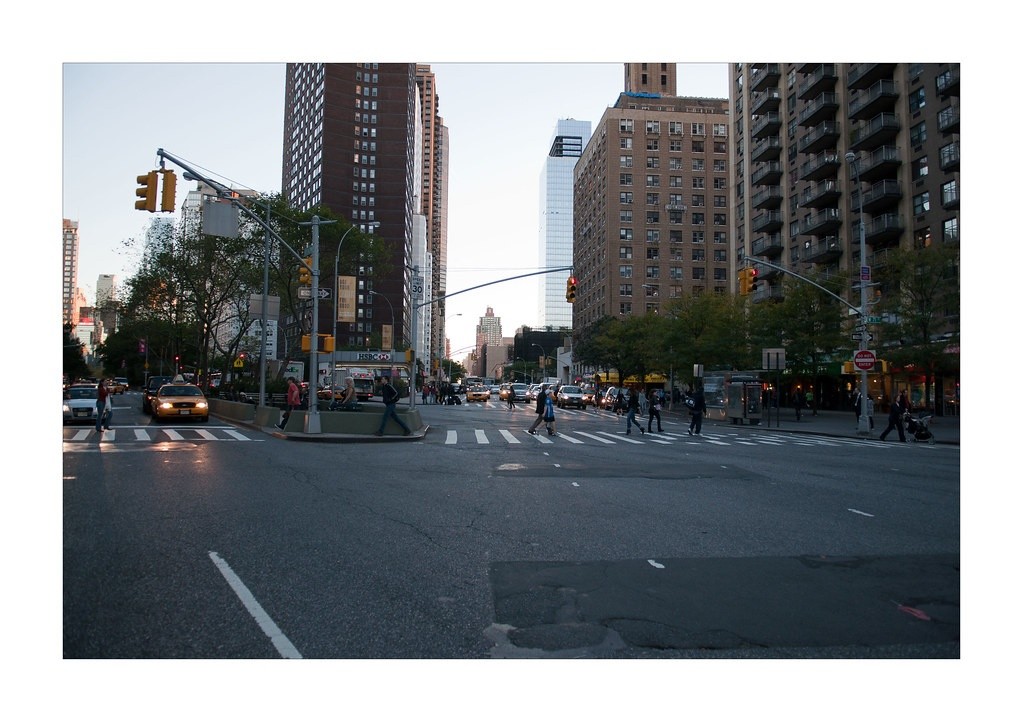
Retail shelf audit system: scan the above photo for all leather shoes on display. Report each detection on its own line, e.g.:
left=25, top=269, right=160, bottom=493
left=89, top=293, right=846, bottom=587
left=96, top=428, right=104, bottom=433
left=104, top=426, right=112, bottom=430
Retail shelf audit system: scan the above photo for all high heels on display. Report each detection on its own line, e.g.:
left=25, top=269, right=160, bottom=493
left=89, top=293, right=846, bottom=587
left=640, top=427, right=646, bottom=436
left=624, top=429, right=632, bottom=437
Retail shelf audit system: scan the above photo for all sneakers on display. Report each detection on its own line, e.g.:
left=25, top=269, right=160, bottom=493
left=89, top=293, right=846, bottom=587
left=274, top=423, right=284, bottom=431
left=372, top=429, right=383, bottom=436
left=403, top=430, right=413, bottom=436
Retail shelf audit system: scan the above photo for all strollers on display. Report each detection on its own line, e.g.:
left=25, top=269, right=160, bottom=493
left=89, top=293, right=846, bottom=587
left=905, top=410, right=936, bottom=446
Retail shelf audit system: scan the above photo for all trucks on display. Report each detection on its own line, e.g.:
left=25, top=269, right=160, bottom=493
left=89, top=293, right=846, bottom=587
left=326, top=367, right=376, bottom=401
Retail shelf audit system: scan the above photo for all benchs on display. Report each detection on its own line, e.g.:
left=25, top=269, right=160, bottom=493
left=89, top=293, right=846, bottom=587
left=244, top=392, right=271, bottom=404
left=272, top=393, right=287, bottom=403
left=218, top=391, right=239, bottom=401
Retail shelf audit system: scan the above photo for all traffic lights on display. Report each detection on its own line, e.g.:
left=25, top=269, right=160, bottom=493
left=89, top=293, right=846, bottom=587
left=746, top=268, right=759, bottom=293
left=406, top=349, right=416, bottom=362
left=134, top=172, right=157, bottom=213
left=567, top=277, right=576, bottom=302
left=299, top=256, right=315, bottom=285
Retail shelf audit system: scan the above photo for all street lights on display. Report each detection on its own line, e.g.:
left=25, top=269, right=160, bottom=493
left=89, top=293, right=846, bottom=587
left=517, top=327, right=575, bottom=388
left=438, top=312, right=463, bottom=379
left=332, top=220, right=380, bottom=408
left=182, top=171, right=272, bottom=406
left=368, top=290, right=394, bottom=365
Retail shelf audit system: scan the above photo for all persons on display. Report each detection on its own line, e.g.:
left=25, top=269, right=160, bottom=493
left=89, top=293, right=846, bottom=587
left=274, top=377, right=303, bottom=430
left=648, top=392, right=664, bottom=432
left=528, top=386, right=553, bottom=435
left=591, top=388, right=681, bottom=416
left=372, top=375, right=412, bottom=437
left=761, top=389, right=773, bottom=414
left=95, top=378, right=114, bottom=432
left=421, top=382, right=463, bottom=405
left=341, top=377, right=358, bottom=412
left=625, top=388, right=646, bottom=435
left=541, top=389, right=557, bottom=436
left=688, top=387, right=706, bottom=436
left=879, top=394, right=908, bottom=442
left=867, top=394, right=875, bottom=430
left=508, top=386, right=516, bottom=409
left=853, top=387, right=862, bottom=422
left=554, top=386, right=560, bottom=399
left=893, top=389, right=912, bottom=430
left=806, top=392, right=813, bottom=410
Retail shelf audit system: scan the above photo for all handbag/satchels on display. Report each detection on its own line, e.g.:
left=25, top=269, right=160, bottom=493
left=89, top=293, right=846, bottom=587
left=654, top=398, right=663, bottom=411
left=684, top=392, right=698, bottom=409
left=102, top=396, right=112, bottom=414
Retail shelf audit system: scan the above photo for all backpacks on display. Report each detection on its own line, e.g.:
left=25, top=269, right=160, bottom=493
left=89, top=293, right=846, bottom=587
left=386, top=383, right=400, bottom=403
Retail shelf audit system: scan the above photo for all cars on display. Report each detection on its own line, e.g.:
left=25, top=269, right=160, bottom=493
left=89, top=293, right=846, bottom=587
left=63, top=387, right=111, bottom=423
left=152, top=374, right=209, bottom=422
left=427, top=375, right=686, bottom=416
left=63, top=375, right=130, bottom=396
left=316, top=386, right=347, bottom=400
left=141, top=376, right=176, bottom=414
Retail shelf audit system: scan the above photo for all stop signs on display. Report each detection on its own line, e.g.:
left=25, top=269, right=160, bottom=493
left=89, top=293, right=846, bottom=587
left=854, top=350, right=876, bottom=371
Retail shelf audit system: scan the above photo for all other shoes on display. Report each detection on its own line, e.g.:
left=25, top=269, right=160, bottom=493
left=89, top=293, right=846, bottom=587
left=688, top=428, right=694, bottom=437
left=658, top=427, right=664, bottom=433
left=647, top=430, right=654, bottom=434
